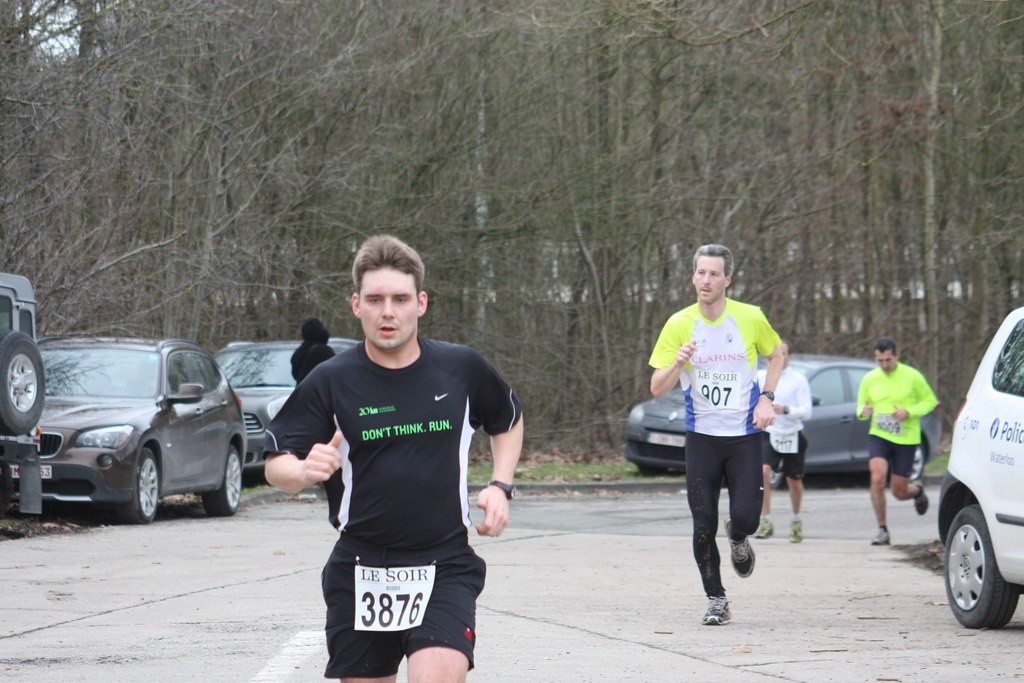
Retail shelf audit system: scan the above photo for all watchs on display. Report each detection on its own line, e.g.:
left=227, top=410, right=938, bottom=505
left=762, top=392, right=774, bottom=401
left=488, top=480, right=516, bottom=500
left=784, top=406, right=790, bottom=414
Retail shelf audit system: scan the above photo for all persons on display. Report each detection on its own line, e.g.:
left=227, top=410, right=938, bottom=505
left=648, top=245, right=785, bottom=625
left=291, top=318, right=345, bottom=532
left=262, top=234, right=524, bottom=683
left=856, top=340, right=939, bottom=545
left=754, top=339, right=812, bottom=543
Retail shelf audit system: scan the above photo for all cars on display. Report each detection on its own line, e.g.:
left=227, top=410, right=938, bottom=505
left=937, top=306, right=1024, bottom=629
left=624, top=353, right=943, bottom=490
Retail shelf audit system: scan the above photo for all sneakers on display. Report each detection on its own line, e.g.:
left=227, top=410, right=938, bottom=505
left=722, top=517, right=755, bottom=578
left=753, top=519, right=774, bottom=538
left=789, top=520, right=804, bottom=543
left=700, top=595, right=733, bottom=625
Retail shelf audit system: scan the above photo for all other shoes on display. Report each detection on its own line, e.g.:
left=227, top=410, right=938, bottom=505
left=870, top=528, right=890, bottom=545
left=913, top=483, right=928, bottom=516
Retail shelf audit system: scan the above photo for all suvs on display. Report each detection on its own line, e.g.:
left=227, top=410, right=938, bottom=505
left=0, top=273, right=46, bottom=523
left=36, top=336, right=248, bottom=525
left=215, top=338, right=359, bottom=489
